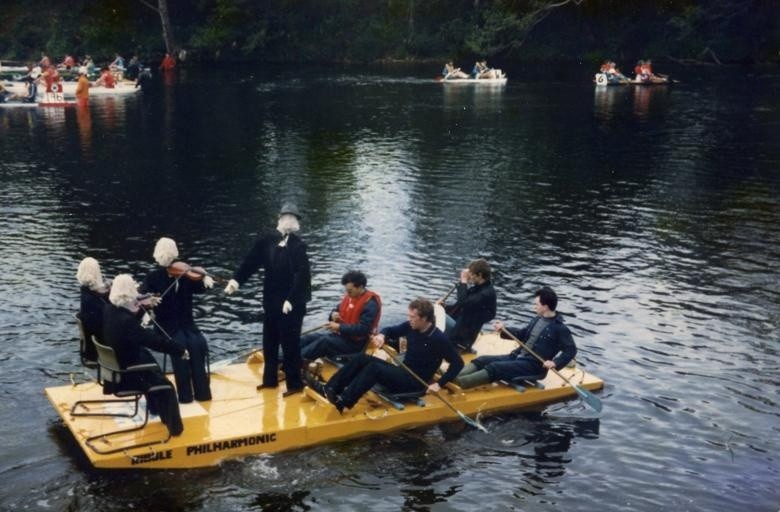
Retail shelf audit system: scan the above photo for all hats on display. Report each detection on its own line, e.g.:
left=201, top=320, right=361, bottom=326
left=279, top=201, right=304, bottom=222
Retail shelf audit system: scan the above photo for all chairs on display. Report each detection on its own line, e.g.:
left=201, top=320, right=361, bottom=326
left=68, top=312, right=212, bottom=454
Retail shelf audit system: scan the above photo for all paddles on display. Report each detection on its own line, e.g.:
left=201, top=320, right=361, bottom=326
left=205, top=326, right=324, bottom=371
left=383, top=345, right=484, bottom=430
left=501, top=327, right=602, bottom=413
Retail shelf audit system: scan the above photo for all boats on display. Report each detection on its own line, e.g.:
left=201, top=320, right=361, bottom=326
left=43, top=329, right=604, bottom=469
left=0, top=58, right=152, bottom=107
left=436, top=77, right=508, bottom=83
left=592, top=77, right=680, bottom=85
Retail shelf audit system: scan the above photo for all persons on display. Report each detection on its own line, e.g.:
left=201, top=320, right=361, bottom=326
left=100, top=273, right=190, bottom=438
left=441, top=57, right=506, bottom=80
left=136, top=236, right=220, bottom=402
left=276, top=270, right=382, bottom=381
left=376, top=258, right=497, bottom=351
left=76, top=257, right=111, bottom=362
left=304, top=297, right=465, bottom=415
left=598, top=57, right=666, bottom=82
left=223, top=202, right=313, bottom=391
left=435, top=286, right=578, bottom=395
left=20, top=51, right=177, bottom=111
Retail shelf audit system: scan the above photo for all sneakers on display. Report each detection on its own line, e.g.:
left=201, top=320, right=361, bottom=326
left=310, top=377, right=339, bottom=404
left=321, top=384, right=347, bottom=414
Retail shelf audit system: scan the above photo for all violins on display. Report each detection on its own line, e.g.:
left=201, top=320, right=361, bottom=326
left=132, top=293, right=161, bottom=313
left=167, top=261, right=224, bottom=283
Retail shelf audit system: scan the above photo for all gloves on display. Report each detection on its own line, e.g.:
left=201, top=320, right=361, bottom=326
left=281, top=298, right=296, bottom=317
left=223, top=279, right=242, bottom=297
